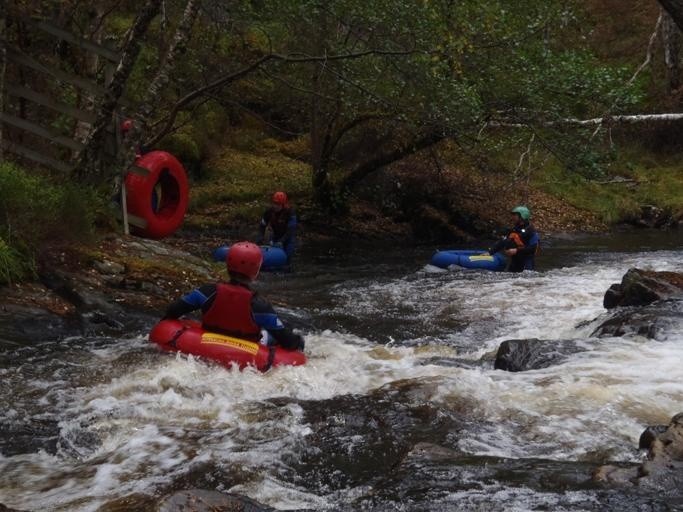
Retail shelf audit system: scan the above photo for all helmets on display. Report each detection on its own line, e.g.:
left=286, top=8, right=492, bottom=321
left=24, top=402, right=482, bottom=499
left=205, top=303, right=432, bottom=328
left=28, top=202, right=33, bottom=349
left=226, top=241, right=264, bottom=282
left=511, top=206, right=530, bottom=221
left=272, top=191, right=287, bottom=204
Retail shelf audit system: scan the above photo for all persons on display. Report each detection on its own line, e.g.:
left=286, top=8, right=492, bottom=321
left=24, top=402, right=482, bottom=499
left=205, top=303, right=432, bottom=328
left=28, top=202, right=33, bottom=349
left=488, top=206, right=538, bottom=272
left=256, top=192, right=297, bottom=262
left=122, top=120, right=141, bottom=158
left=160, top=241, right=304, bottom=352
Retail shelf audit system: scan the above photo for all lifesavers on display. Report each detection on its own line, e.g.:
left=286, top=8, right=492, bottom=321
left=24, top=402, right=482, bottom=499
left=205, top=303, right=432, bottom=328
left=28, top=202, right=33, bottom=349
left=432, top=248, right=503, bottom=272
left=214, top=243, right=289, bottom=270
left=149, top=317, right=306, bottom=373
left=122, top=150, right=188, bottom=240
